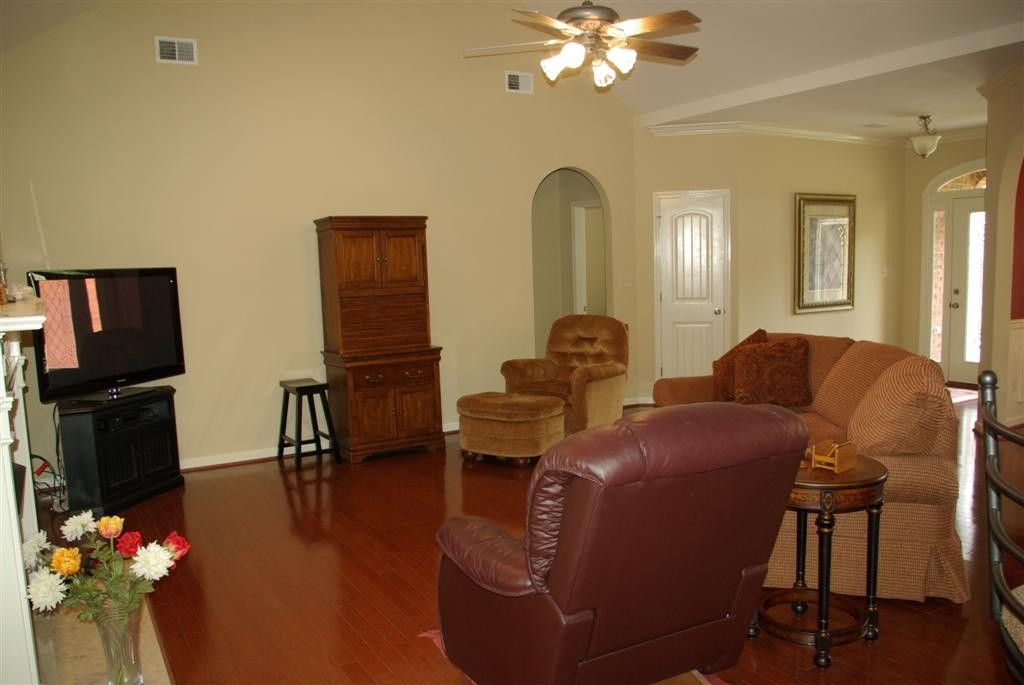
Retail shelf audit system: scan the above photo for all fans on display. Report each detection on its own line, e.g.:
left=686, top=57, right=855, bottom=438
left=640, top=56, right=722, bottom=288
left=462, top=1, right=702, bottom=62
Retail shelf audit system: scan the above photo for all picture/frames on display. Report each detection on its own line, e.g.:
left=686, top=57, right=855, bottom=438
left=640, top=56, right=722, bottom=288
left=794, top=192, right=857, bottom=314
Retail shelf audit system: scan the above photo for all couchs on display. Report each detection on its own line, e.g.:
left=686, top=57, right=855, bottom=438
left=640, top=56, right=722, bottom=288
left=434, top=403, right=810, bottom=685
left=501, top=314, right=631, bottom=438
left=653, top=328, right=972, bottom=604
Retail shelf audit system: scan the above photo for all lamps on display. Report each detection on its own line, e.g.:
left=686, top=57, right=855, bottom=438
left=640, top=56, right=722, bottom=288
left=539, top=36, right=638, bottom=87
left=907, top=116, right=942, bottom=160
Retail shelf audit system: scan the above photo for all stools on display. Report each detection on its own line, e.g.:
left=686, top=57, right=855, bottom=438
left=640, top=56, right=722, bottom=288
left=457, top=391, right=566, bottom=468
left=277, top=378, right=341, bottom=473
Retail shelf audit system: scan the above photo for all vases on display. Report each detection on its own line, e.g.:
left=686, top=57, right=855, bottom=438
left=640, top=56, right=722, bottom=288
left=93, top=607, right=142, bottom=684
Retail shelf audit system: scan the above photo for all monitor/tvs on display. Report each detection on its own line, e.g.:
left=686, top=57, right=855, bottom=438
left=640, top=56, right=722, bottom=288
left=26, top=266, right=186, bottom=403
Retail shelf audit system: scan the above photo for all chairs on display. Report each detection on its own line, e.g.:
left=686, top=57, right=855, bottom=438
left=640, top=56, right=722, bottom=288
left=977, top=370, right=1024, bottom=685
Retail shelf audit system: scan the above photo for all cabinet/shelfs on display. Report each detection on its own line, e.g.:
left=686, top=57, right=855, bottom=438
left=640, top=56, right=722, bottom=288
left=59, top=385, right=184, bottom=521
left=314, top=215, right=447, bottom=464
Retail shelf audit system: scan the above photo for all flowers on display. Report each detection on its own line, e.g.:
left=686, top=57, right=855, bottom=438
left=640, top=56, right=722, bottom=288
left=21, top=509, right=192, bottom=684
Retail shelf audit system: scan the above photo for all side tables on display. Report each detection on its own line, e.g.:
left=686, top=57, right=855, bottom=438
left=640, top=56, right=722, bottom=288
left=747, top=455, right=888, bottom=670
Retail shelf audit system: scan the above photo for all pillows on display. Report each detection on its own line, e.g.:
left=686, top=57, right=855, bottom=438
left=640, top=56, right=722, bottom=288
left=713, top=328, right=769, bottom=403
left=734, top=336, right=812, bottom=408
